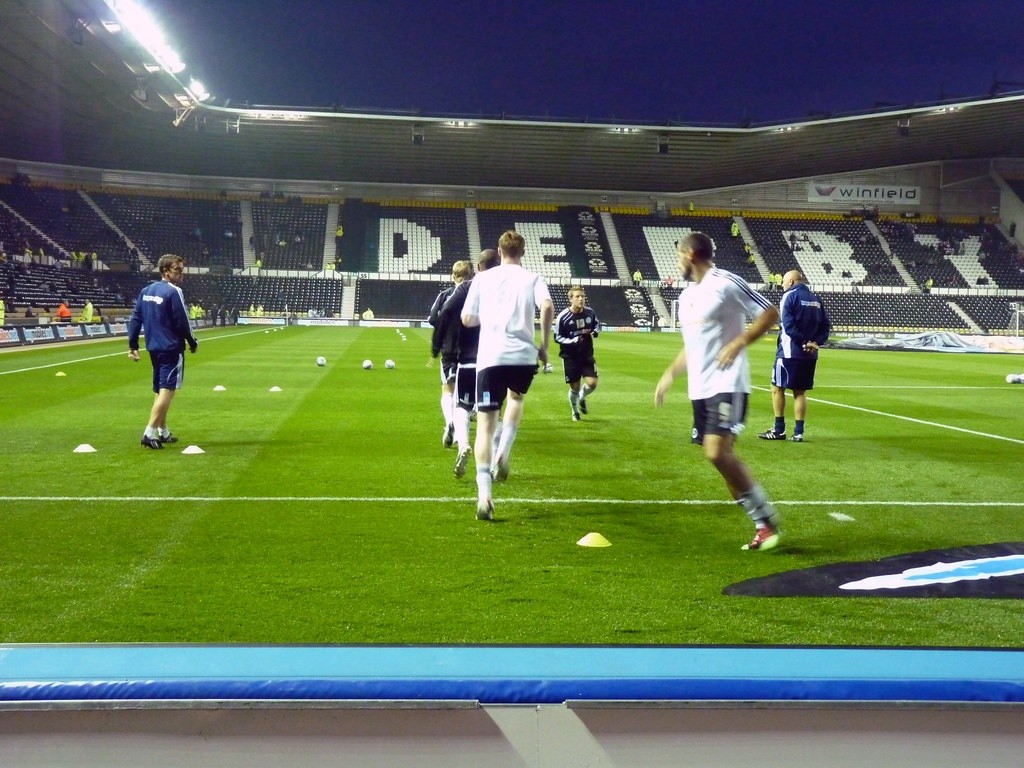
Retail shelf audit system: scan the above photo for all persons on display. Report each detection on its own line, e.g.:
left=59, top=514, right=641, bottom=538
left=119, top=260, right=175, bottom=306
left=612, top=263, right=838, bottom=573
left=653, top=232, right=785, bottom=550
left=755, top=270, right=830, bottom=441
left=428, top=248, right=505, bottom=483
left=0, top=242, right=99, bottom=327
left=182, top=222, right=345, bottom=327
left=461, top=231, right=554, bottom=520
left=633, top=201, right=1024, bottom=295
left=553, top=285, right=600, bottom=422
left=128, top=254, right=198, bottom=449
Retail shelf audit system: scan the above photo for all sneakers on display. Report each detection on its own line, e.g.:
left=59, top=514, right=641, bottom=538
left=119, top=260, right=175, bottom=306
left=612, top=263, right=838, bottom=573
left=758, top=428, right=787, bottom=440
left=741, top=521, right=780, bottom=550
left=792, top=434, right=803, bottom=442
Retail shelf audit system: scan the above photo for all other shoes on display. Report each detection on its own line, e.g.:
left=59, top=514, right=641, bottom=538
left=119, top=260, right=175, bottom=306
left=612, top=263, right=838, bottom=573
left=443, top=422, right=455, bottom=448
left=494, top=455, right=509, bottom=481
left=159, top=433, right=179, bottom=443
left=454, top=445, right=472, bottom=479
left=572, top=412, right=580, bottom=421
left=140, top=434, right=162, bottom=449
left=476, top=497, right=494, bottom=520
left=578, top=399, right=587, bottom=414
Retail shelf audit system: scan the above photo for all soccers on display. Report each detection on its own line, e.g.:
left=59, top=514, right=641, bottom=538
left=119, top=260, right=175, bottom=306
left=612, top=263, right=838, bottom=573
left=543, top=363, right=554, bottom=373
left=317, top=357, right=326, bottom=366
left=363, top=360, right=373, bottom=370
left=385, top=359, right=395, bottom=368
